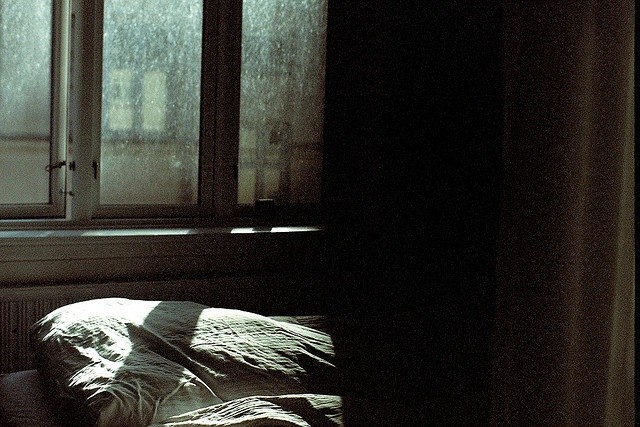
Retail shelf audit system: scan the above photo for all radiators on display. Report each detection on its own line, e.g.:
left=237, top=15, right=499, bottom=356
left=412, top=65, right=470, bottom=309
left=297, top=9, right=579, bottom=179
left=0, top=274, right=270, bottom=374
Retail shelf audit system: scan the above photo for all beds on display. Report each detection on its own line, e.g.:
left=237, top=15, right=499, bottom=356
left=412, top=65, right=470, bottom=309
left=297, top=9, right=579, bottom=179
left=0, top=296, right=344, bottom=427
